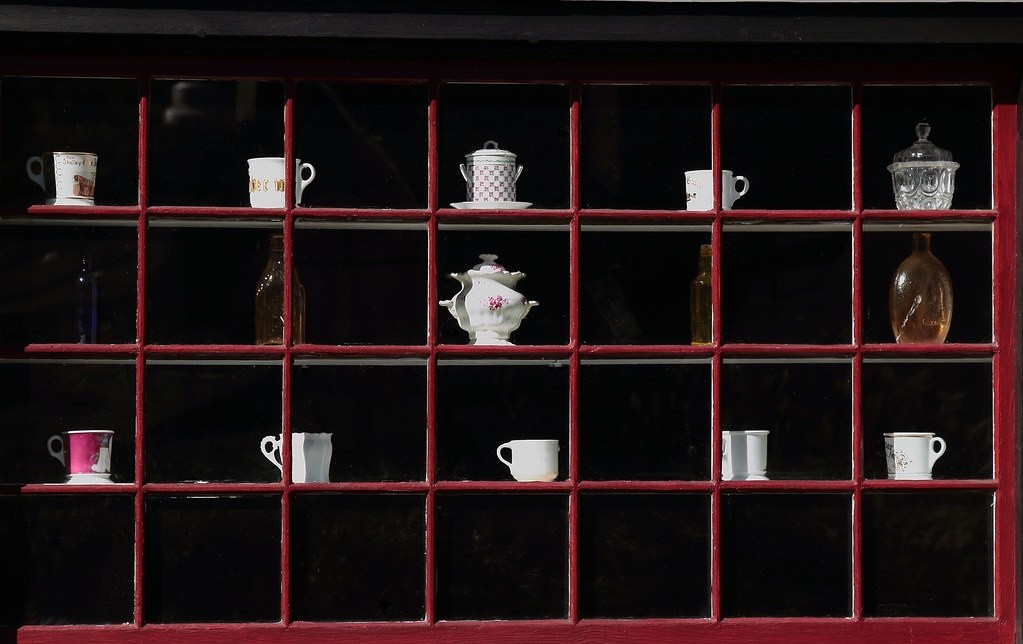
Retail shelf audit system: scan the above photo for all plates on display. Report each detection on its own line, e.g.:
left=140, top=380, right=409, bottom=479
left=450, top=201, right=533, bottom=210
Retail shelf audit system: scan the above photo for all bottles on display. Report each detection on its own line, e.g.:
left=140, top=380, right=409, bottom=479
left=254, top=235, right=305, bottom=345
left=438, top=254, right=539, bottom=345
left=68, top=255, right=97, bottom=345
left=890, top=233, right=953, bottom=344
left=691, top=243, right=722, bottom=345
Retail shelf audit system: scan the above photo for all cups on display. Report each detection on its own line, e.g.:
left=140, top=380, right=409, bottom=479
left=259, top=432, right=333, bottom=484
left=247, top=157, right=315, bottom=208
left=26, top=152, right=99, bottom=207
left=459, top=140, right=523, bottom=201
left=883, top=432, right=947, bottom=481
left=48, top=430, right=115, bottom=482
left=886, top=122, right=960, bottom=210
left=721, top=429, right=772, bottom=481
left=496, top=439, right=559, bottom=482
left=684, top=170, right=749, bottom=211
